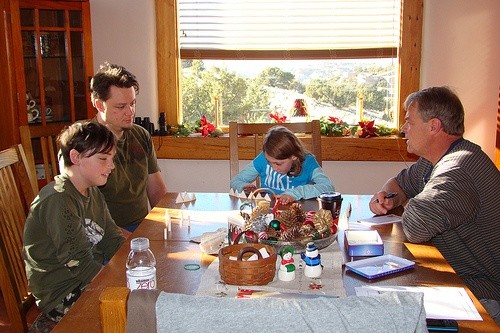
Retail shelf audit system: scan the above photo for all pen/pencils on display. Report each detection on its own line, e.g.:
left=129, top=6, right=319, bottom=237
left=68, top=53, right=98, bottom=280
left=371, top=192, right=398, bottom=204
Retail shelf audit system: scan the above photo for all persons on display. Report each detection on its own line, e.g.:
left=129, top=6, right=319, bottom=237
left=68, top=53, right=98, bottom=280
left=230, top=126, right=335, bottom=204
left=22, top=120, right=127, bottom=322
left=369, top=87, right=500, bottom=323
left=90, top=60, right=167, bottom=239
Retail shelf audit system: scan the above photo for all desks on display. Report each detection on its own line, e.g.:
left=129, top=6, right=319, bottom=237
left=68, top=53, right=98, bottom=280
left=47, top=193, right=500, bottom=333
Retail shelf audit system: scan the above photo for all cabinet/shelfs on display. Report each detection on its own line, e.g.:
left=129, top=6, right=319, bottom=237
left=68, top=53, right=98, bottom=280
left=0, top=0, right=95, bottom=143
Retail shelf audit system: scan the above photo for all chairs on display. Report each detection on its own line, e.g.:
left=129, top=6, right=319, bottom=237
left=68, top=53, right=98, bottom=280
left=229, top=120, right=326, bottom=196
left=22, top=126, right=66, bottom=191
left=0, top=146, right=38, bottom=333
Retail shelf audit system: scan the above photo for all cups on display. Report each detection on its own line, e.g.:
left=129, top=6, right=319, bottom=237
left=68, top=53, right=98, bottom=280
left=39, top=106, right=52, bottom=116
left=316, top=191, right=344, bottom=235
left=26, top=91, right=40, bottom=123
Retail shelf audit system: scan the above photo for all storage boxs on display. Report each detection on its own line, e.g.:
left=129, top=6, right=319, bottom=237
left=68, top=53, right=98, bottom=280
left=344, top=230, right=385, bottom=255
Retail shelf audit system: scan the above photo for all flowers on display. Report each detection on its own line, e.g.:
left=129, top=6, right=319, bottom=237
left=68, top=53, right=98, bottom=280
left=178, top=98, right=397, bottom=138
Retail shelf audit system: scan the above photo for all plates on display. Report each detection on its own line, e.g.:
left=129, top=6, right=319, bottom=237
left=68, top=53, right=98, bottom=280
left=37, top=120, right=55, bottom=122
left=37, top=115, right=52, bottom=120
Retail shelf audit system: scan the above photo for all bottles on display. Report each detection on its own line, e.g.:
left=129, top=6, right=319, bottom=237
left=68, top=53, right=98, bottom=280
left=125, top=237, right=157, bottom=290
